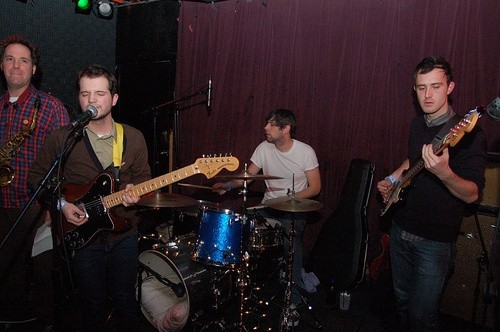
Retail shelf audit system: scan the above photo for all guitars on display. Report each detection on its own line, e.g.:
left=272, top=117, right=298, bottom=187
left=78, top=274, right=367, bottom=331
left=375, top=93, right=500, bottom=217
left=56, top=152, right=239, bottom=251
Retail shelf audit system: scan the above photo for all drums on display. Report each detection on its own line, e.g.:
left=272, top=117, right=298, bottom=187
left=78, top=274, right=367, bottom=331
left=133, top=233, right=229, bottom=332
left=192, top=201, right=247, bottom=272
left=244, top=206, right=284, bottom=251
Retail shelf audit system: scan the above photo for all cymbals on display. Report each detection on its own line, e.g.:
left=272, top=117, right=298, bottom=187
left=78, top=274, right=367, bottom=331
left=210, top=174, right=285, bottom=182
left=134, top=192, right=199, bottom=207
left=264, top=196, right=324, bottom=213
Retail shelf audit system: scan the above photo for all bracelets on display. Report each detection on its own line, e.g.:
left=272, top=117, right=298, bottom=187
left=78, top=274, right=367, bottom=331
left=386, top=175, right=397, bottom=187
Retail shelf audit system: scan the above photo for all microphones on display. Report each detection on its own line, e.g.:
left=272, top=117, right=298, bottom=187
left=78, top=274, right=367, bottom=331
left=65, top=106, right=98, bottom=131
left=208, top=76, right=212, bottom=107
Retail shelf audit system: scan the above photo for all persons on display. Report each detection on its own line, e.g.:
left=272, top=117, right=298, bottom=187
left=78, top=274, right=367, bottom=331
left=211, top=108, right=322, bottom=328
left=0, top=35, right=71, bottom=332
left=26, top=63, right=153, bottom=332
left=375, top=53, right=488, bottom=331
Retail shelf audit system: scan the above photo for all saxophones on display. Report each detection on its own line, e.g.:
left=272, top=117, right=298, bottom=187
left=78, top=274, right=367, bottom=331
left=1, top=96, right=41, bottom=185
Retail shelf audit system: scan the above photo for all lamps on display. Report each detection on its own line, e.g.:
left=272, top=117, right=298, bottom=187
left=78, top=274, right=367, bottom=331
left=97, top=2, right=114, bottom=20
left=74, top=0, right=93, bottom=16
